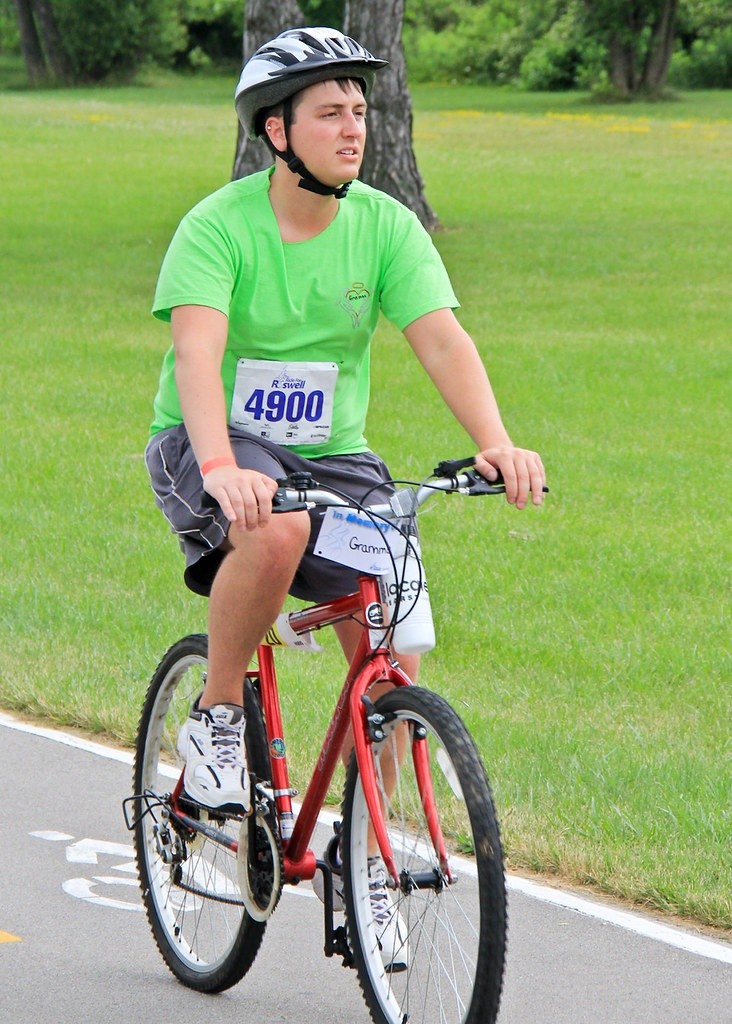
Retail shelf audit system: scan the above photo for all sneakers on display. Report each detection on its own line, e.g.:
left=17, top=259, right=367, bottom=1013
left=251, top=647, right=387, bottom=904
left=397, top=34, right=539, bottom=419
left=313, top=839, right=416, bottom=972
left=180, top=700, right=253, bottom=817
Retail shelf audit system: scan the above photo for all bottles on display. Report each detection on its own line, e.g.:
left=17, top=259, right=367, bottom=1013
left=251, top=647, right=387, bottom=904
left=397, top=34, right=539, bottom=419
left=378, top=523, right=436, bottom=656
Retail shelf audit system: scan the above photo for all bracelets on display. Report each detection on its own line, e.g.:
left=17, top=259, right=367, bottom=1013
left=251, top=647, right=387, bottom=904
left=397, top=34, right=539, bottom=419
left=198, top=457, right=236, bottom=478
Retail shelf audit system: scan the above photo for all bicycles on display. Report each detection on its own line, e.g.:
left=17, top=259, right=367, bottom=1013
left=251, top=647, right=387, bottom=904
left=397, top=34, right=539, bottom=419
left=114, top=449, right=553, bottom=1023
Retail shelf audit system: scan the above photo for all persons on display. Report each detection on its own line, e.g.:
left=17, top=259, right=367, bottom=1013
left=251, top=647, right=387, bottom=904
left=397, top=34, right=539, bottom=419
left=145, top=25, right=545, bottom=973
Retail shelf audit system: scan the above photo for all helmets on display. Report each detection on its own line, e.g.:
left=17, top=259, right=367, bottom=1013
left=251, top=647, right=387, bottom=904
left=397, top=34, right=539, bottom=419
left=233, top=23, right=389, bottom=144
